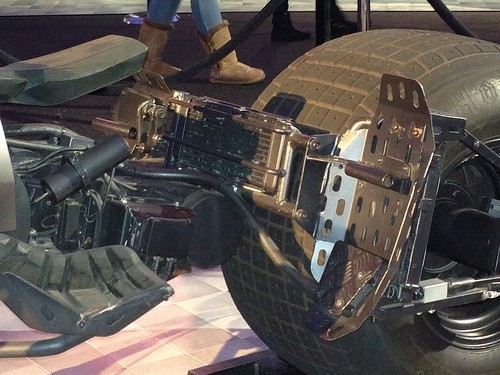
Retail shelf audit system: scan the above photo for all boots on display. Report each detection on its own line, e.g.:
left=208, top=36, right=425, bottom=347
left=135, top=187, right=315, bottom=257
left=272, top=11, right=310, bottom=42
left=138, top=17, right=181, bottom=78
left=332, top=6, right=359, bottom=36
left=197, top=19, right=265, bottom=85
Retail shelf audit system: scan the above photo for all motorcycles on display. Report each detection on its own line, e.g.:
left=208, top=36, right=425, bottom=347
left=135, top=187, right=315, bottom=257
left=0, top=28, right=500, bottom=375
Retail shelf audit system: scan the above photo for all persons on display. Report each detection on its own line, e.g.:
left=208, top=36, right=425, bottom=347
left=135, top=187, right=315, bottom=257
left=136, top=0, right=265, bottom=85
left=268, top=0, right=362, bottom=42
